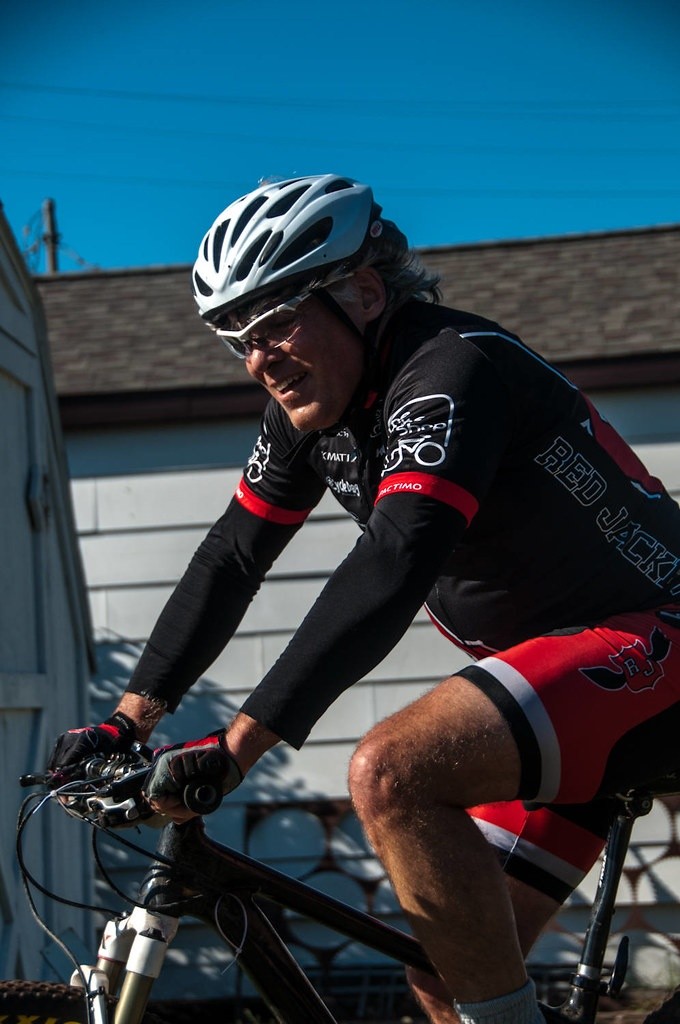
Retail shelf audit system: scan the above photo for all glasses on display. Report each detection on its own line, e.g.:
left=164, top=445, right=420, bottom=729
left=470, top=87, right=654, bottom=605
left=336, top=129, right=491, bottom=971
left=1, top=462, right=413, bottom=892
left=219, top=279, right=346, bottom=360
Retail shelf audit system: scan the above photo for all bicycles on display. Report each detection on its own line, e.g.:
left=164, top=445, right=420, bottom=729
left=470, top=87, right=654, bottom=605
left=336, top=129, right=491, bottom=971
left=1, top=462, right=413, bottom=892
left=1, top=679, right=680, bottom=1024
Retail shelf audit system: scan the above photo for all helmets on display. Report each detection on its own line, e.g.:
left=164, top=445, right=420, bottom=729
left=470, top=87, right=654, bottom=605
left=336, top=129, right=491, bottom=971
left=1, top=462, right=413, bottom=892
left=191, top=173, right=375, bottom=322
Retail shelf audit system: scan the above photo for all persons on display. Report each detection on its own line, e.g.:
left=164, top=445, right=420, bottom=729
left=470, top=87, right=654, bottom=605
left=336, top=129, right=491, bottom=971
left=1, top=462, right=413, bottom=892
left=47, top=175, right=680, bottom=1024
